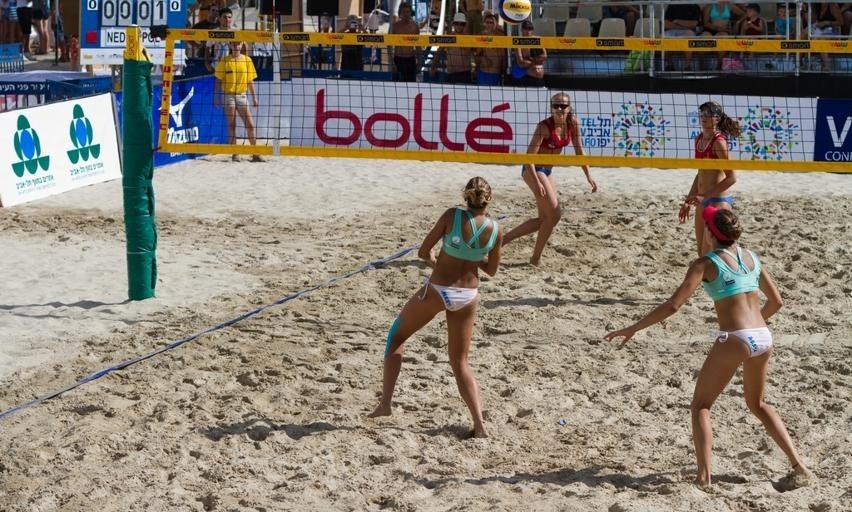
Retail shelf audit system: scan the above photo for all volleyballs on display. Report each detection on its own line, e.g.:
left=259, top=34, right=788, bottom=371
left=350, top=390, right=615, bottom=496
left=498, top=0, right=531, bottom=24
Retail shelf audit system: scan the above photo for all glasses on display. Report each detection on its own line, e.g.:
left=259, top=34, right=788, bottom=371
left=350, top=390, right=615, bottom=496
left=552, top=104, right=569, bottom=109
left=700, top=111, right=714, bottom=117
left=524, top=27, right=533, bottom=30
left=486, top=21, right=494, bottom=24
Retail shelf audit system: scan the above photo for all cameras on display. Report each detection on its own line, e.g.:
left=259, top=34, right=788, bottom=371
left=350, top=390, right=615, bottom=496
left=349, top=21, right=360, bottom=29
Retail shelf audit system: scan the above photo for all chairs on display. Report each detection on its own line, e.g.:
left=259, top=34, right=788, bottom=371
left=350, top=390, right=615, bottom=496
left=457, top=0, right=852, bottom=37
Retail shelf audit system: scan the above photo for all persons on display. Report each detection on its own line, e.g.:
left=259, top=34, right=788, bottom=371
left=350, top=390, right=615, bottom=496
left=602, top=0, right=852, bottom=76
left=603, top=209, right=813, bottom=492
left=367, top=177, right=503, bottom=439
left=501, top=92, right=598, bottom=268
left=336, top=0, right=549, bottom=86
left=679, top=100, right=742, bottom=263
left=213, top=41, right=268, bottom=162
left=0, top=0, right=71, bottom=63
left=185, top=0, right=249, bottom=76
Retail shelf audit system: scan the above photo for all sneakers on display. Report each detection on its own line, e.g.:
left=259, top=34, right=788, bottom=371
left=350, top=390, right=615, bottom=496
left=232, top=155, right=241, bottom=161
left=253, top=155, right=267, bottom=162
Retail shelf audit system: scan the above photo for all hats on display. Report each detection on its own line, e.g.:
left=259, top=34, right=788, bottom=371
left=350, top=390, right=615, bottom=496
left=521, top=21, right=533, bottom=27
left=699, top=102, right=722, bottom=116
left=701, top=206, right=731, bottom=241
left=452, top=13, right=467, bottom=25
left=346, top=15, right=358, bottom=24
